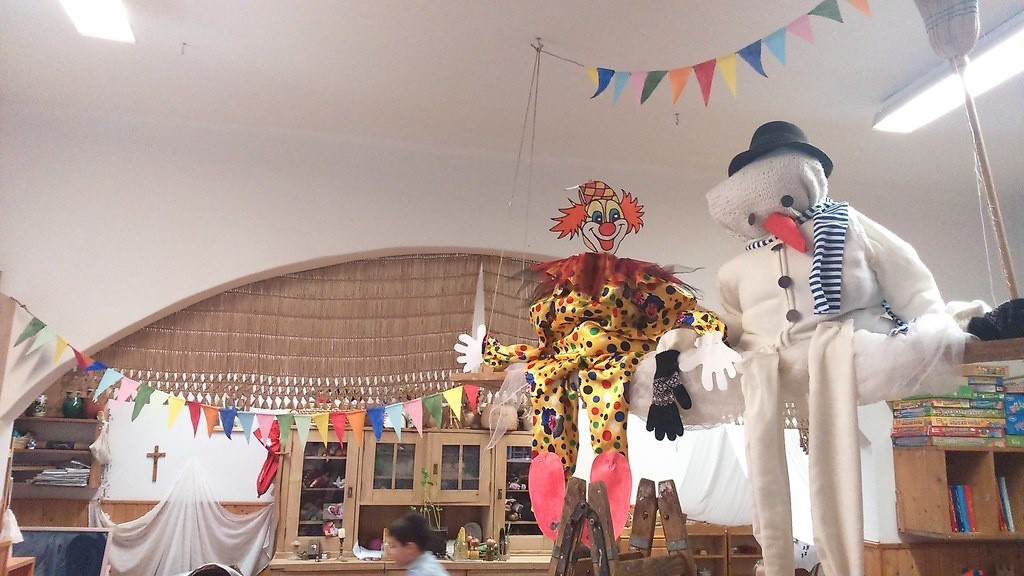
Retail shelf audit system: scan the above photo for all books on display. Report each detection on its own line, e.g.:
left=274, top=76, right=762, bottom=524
left=949, top=476, right=978, bottom=532
left=994, top=475, right=1016, bottom=533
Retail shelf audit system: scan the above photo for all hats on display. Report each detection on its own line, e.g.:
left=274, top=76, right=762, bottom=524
left=727, top=121, right=834, bottom=178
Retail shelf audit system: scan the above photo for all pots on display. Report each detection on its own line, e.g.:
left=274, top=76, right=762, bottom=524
left=481, top=403, right=519, bottom=430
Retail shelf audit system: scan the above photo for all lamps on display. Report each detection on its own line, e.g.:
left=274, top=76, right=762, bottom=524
left=872, top=10, right=1024, bottom=133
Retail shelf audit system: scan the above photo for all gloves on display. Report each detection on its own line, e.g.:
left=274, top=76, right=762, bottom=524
left=645, top=348, right=694, bottom=443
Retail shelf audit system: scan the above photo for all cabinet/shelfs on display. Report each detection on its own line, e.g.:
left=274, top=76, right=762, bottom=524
left=360, top=424, right=491, bottom=505
left=8, top=407, right=103, bottom=490
left=617, top=526, right=687, bottom=561
left=891, top=336, right=1024, bottom=542
left=687, top=524, right=727, bottom=576
left=493, top=431, right=564, bottom=555
left=273, top=423, right=361, bottom=557
left=727, top=525, right=763, bottom=576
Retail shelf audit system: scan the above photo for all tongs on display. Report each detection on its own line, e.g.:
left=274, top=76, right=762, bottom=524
left=499, top=528, right=506, bottom=555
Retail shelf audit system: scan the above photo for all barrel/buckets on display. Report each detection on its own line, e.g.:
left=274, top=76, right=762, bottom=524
left=496, top=540, right=511, bottom=561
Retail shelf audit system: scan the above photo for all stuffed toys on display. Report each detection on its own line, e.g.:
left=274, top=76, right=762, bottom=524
left=641, top=120, right=978, bottom=576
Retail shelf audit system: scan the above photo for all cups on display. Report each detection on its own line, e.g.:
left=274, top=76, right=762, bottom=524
left=328, top=503, right=343, bottom=519
left=380, top=543, right=390, bottom=561
left=462, top=409, right=476, bottom=428
left=454, top=542, right=468, bottom=560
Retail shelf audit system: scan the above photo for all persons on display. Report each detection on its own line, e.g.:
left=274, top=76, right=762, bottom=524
left=387, top=511, right=450, bottom=576
left=0, top=507, right=24, bottom=576
